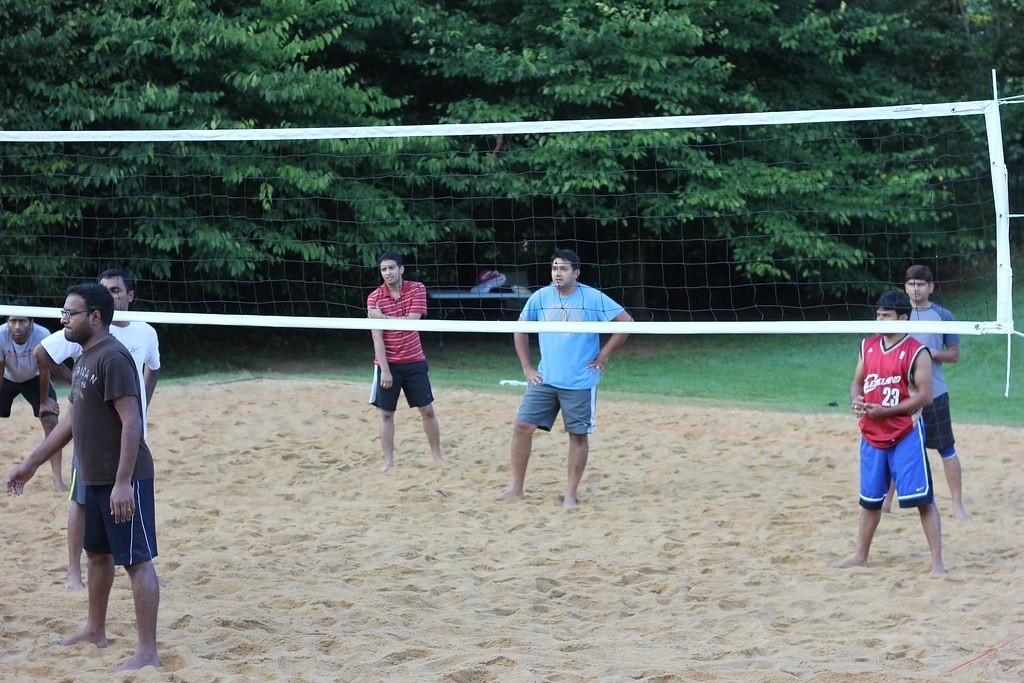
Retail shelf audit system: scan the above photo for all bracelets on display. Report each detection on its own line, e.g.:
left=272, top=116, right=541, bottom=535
left=386, top=314, right=387, bottom=319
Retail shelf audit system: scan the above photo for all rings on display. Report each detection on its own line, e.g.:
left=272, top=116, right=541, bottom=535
left=854, top=405, right=859, bottom=410
left=596, top=366, right=599, bottom=369
left=127, top=508, right=132, bottom=511
left=383, top=384, right=386, bottom=386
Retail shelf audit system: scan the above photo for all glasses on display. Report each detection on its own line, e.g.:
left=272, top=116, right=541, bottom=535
left=61, top=308, right=103, bottom=317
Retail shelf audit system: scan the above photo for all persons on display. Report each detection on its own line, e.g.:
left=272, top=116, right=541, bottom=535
left=496, top=249, right=635, bottom=511
left=0, top=299, right=69, bottom=494
left=905, top=264, right=966, bottom=520
left=52, top=283, right=164, bottom=672
left=31, top=267, right=161, bottom=590
left=831, top=289, right=946, bottom=580
left=366, top=252, right=446, bottom=473
